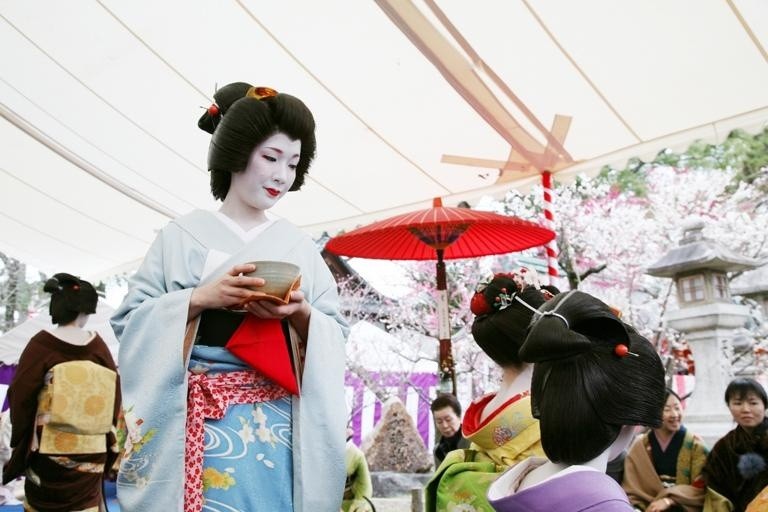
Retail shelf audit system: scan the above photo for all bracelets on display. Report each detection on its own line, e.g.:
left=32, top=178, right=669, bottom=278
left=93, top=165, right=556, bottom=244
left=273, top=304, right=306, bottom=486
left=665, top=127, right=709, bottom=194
left=662, top=497, right=671, bottom=508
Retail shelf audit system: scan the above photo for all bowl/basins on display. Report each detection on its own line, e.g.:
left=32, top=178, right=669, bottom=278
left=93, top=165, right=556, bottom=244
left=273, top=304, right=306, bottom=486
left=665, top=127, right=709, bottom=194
left=242, top=260, right=300, bottom=297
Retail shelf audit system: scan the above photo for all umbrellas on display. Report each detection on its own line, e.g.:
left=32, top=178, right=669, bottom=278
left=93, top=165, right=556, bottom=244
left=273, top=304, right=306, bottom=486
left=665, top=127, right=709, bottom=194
left=323, top=197, right=556, bottom=378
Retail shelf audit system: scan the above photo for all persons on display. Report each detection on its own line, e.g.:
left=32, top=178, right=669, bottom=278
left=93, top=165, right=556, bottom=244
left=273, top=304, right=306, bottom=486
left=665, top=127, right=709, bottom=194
left=701, top=378, right=768, bottom=512
left=109, top=80, right=350, bottom=512
left=485, top=289, right=666, bottom=511
left=431, top=395, right=473, bottom=472
left=622, top=386, right=708, bottom=512
left=460, top=273, right=560, bottom=468
left=3, top=272, right=122, bottom=512
